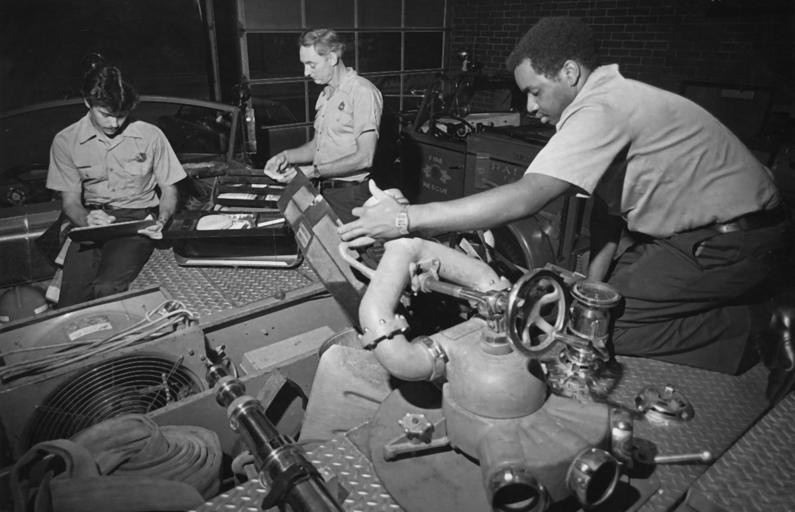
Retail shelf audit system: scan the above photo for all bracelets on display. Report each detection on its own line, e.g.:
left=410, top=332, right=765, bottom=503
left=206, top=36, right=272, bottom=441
left=159, top=217, right=167, bottom=222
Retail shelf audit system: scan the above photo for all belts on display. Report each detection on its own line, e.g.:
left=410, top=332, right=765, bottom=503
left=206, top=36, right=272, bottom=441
left=321, top=175, right=373, bottom=191
left=715, top=200, right=787, bottom=234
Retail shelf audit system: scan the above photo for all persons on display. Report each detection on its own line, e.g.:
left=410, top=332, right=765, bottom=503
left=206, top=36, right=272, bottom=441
left=262, top=24, right=384, bottom=225
left=44, top=67, right=189, bottom=309
left=335, top=14, right=795, bottom=377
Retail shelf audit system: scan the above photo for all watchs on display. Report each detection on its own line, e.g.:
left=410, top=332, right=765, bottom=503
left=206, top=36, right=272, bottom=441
left=314, top=164, right=321, bottom=179
left=396, top=202, right=409, bottom=236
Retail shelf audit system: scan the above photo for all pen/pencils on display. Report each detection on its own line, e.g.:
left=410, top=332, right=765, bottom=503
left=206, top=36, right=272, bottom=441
left=102, top=204, right=107, bottom=214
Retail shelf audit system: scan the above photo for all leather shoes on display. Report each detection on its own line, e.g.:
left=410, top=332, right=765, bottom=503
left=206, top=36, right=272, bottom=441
left=749, top=290, right=794, bottom=404
